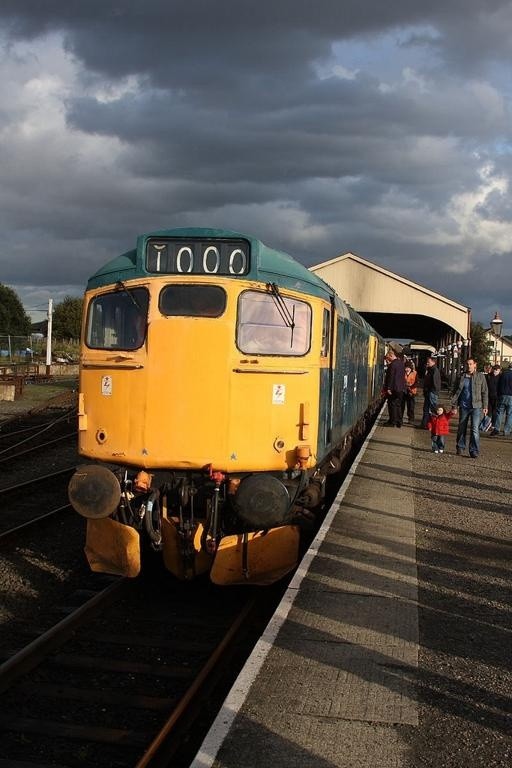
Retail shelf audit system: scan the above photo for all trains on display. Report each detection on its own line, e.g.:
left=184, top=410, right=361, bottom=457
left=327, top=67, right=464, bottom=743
left=67, top=227, right=390, bottom=590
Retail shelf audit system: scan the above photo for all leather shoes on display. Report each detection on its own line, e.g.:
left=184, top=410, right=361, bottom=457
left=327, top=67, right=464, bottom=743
left=384, top=422, right=401, bottom=428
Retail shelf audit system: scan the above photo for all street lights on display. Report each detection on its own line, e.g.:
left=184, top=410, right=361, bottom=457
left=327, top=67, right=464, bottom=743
left=490, top=317, right=503, bottom=366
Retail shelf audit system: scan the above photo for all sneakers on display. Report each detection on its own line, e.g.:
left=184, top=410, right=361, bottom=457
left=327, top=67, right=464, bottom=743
left=456, top=448, right=464, bottom=454
left=490, top=428, right=499, bottom=436
left=470, top=451, right=478, bottom=458
left=434, top=450, right=443, bottom=454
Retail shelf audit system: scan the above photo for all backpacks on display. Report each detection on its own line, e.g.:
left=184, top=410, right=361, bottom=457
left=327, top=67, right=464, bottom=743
left=478, top=416, right=492, bottom=432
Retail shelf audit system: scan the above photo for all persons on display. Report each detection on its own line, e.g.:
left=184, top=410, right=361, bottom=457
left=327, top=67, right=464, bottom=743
left=427, top=404, right=457, bottom=453
left=451, top=358, right=488, bottom=457
left=484, top=362, right=512, bottom=436
left=25, top=346, right=33, bottom=356
left=383, top=351, right=419, bottom=427
left=418, top=356, right=441, bottom=426
